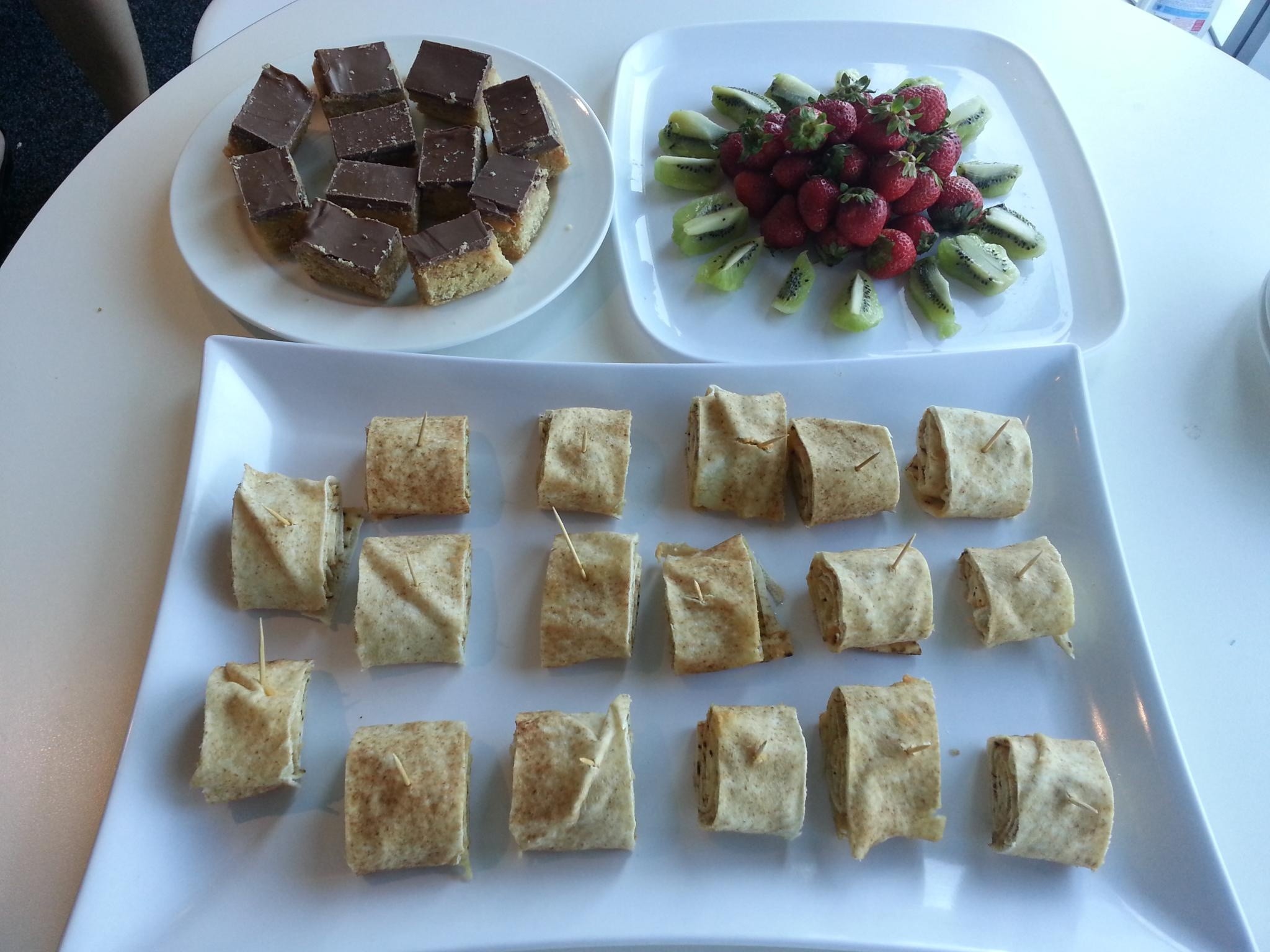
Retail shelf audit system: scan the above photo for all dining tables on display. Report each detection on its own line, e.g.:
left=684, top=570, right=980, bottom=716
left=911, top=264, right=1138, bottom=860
left=2, top=3, right=1270, bottom=952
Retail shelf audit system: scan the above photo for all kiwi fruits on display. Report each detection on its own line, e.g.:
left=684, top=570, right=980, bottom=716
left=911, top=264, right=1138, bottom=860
left=655, top=75, right=1046, bottom=333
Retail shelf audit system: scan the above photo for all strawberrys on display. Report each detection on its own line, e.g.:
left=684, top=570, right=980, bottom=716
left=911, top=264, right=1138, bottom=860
left=717, top=72, right=985, bottom=280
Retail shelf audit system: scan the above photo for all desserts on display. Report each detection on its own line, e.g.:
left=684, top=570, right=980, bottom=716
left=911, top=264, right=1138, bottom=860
left=227, top=39, right=570, bottom=305
left=197, top=381, right=1117, bottom=875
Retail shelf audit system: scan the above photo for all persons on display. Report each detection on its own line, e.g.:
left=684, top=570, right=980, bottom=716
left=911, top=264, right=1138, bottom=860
left=35, top=0, right=149, bottom=119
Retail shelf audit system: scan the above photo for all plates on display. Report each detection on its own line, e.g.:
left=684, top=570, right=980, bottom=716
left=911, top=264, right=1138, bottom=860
left=167, top=32, right=613, bottom=351
left=56, top=323, right=1264, bottom=952
left=610, top=18, right=1126, bottom=365
